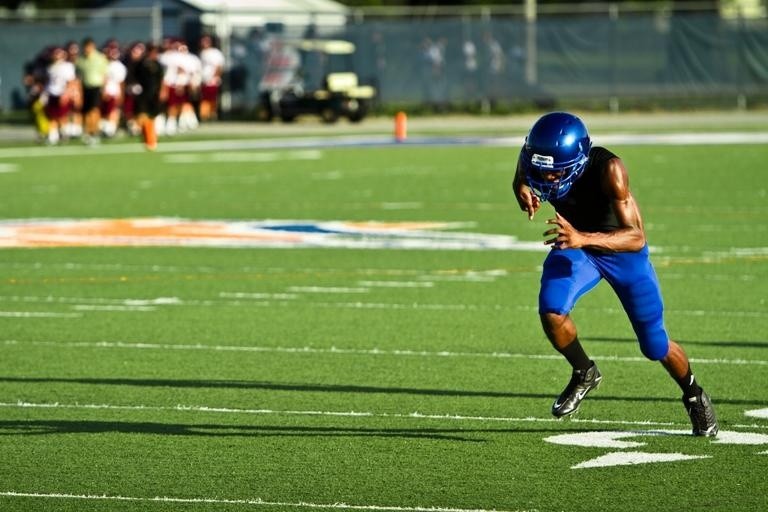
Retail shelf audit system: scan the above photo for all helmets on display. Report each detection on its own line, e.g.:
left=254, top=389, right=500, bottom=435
left=522, top=113, right=591, bottom=200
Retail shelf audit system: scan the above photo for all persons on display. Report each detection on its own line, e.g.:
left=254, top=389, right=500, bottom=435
left=513, top=113, right=718, bottom=438
left=366, top=31, right=508, bottom=100
left=24, top=31, right=227, bottom=146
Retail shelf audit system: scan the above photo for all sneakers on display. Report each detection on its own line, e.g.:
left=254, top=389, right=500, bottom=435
left=682, top=387, right=719, bottom=438
left=552, top=361, right=602, bottom=417
